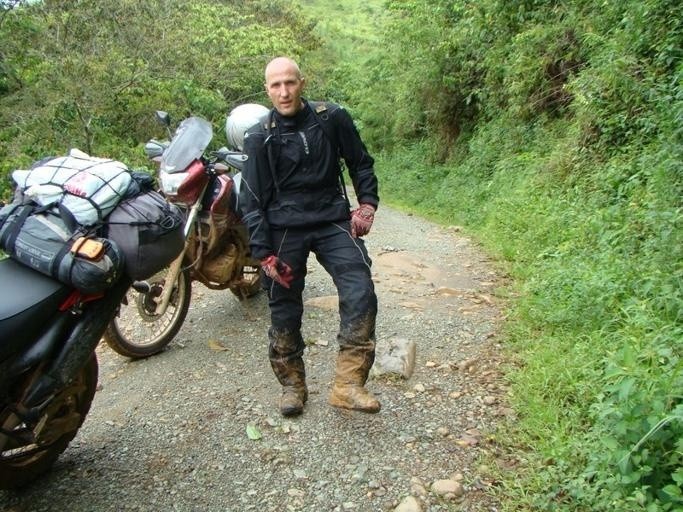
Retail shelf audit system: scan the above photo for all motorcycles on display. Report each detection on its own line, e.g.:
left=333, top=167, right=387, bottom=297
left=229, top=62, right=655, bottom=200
left=1, top=248, right=151, bottom=489
left=105, top=108, right=269, bottom=359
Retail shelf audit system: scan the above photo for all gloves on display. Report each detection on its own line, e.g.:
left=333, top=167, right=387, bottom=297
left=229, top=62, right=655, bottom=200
left=259, top=255, right=295, bottom=289
left=350, top=204, right=375, bottom=239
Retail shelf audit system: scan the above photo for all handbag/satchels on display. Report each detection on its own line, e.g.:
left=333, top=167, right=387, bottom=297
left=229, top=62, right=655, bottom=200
left=13, top=157, right=185, bottom=280
left=0, top=203, right=125, bottom=295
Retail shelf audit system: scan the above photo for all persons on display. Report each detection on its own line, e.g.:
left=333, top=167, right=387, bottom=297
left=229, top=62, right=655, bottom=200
left=237, top=56, right=382, bottom=415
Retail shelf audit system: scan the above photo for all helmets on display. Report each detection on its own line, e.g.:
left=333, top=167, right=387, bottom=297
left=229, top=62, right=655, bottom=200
left=226, top=104, right=270, bottom=152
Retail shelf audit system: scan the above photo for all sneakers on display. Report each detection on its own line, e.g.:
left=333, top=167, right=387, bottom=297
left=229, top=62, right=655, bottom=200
left=329, top=384, right=380, bottom=414
left=280, top=384, right=307, bottom=416
